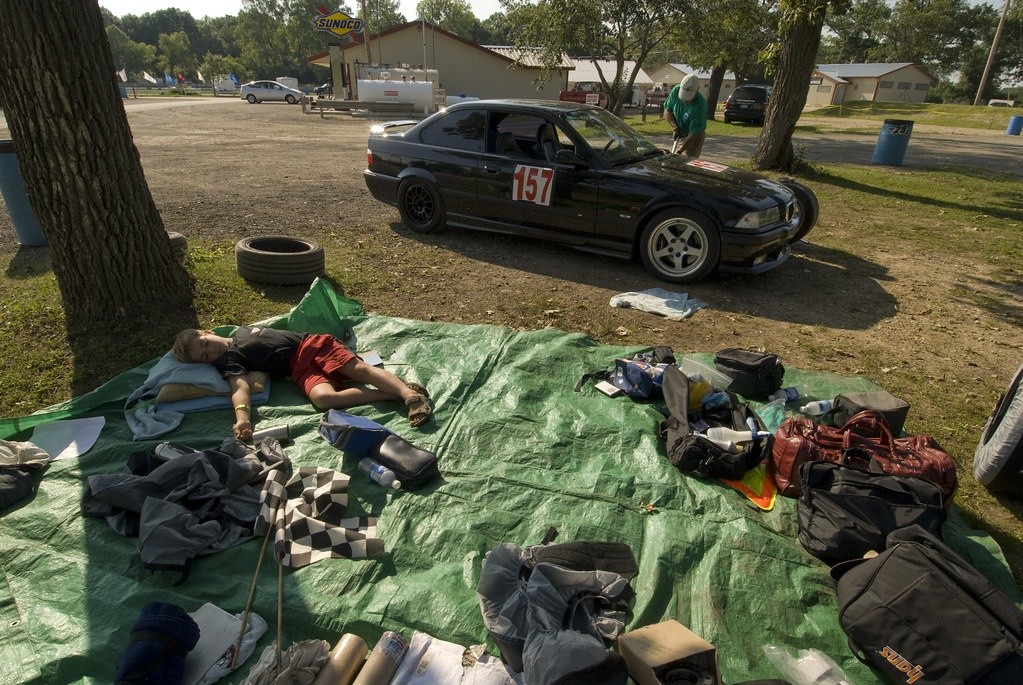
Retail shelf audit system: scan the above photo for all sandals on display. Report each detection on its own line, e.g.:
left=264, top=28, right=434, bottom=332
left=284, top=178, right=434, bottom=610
left=405, top=392, right=432, bottom=427
left=397, top=375, right=430, bottom=398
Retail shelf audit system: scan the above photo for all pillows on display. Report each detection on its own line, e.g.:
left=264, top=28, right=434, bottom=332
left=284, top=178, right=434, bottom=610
left=157, top=371, right=267, bottom=402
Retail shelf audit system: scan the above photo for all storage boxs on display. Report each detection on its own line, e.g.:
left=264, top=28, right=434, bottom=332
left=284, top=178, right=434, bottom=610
left=618, top=619, right=722, bottom=685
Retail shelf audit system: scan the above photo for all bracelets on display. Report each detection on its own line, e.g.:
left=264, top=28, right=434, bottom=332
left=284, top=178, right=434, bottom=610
left=235, top=404, right=251, bottom=410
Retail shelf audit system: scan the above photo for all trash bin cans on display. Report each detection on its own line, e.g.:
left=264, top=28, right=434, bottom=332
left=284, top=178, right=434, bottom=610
left=119, top=87, right=128, bottom=98
left=0, top=139, right=48, bottom=247
left=871, top=119, right=915, bottom=166
left=1006, top=116, right=1023, bottom=135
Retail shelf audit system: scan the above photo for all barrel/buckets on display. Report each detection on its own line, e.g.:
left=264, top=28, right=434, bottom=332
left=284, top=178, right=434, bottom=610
left=0, top=138, right=49, bottom=247
left=872, top=118, right=914, bottom=166
left=1006, top=116, right=1023, bottom=135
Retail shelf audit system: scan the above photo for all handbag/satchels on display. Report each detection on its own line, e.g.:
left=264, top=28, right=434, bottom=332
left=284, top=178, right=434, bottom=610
left=830, top=391, right=910, bottom=437
left=796, top=446, right=946, bottom=562
left=830, top=523, right=1023, bottom=685
left=376, top=432, right=441, bottom=491
left=662, top=364, right=774, bottom=479
left=713, top=347, right=785, bottom=398
left=318, top=408, right=393, bottom=456
left=772, top=409, right=959, bottom=512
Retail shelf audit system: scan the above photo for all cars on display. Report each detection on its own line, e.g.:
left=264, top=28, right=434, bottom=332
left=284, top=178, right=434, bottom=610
left=362, top=99, right=805, bottom=288
left=314, top=84, right=331, bottom=95
left=240, top=79, right=306, bottom=105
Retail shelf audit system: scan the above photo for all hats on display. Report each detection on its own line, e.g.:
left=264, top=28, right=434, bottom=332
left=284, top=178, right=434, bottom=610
left=678, top=73, right=700, bottom=99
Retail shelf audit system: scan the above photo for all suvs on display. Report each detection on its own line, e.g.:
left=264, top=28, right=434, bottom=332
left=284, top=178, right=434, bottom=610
left=723, top=83, right=774, bottom=127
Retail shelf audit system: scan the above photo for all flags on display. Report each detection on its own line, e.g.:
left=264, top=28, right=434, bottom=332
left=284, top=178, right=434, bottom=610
left=230, top=73, right=240, bottom=85
left=118, top=69, right=128, bottom=83
left=143, top=72, right=156, bottom=84
left=178, top=73, right=185, bottom=81
left=165, top=74, right=175, bottom=86
left=197, top=72, right=205, bottom=84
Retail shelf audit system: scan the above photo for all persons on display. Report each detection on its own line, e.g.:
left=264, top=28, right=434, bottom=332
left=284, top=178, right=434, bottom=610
left=662, top=74, right=708, bottom=159
left=172, top=324, right=432, bottom=441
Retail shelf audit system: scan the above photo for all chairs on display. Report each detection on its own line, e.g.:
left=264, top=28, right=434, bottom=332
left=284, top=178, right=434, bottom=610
left=495, top=132, right=531, bottom=158
left=536, top=123, right=563, bottom=161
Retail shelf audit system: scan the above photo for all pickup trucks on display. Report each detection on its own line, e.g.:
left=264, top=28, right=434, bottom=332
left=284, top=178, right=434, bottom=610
left=559, top=81, right=608, bottom=109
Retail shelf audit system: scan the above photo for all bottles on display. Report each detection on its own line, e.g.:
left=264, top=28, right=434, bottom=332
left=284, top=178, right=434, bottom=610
left=358, top=457, right=401, bottom=490
left=800, top=398, right=837, bottom=416
left=768, top=385, right=808, bottom=402
